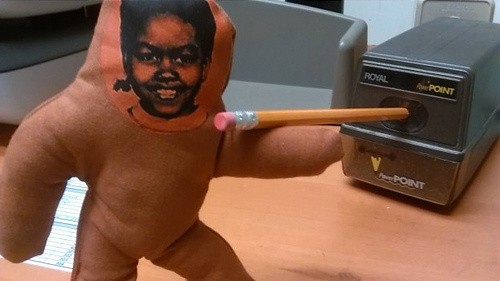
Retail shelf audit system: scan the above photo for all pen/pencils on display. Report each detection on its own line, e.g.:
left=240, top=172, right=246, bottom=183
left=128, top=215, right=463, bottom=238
left=213, top=107, right=413, bottom=132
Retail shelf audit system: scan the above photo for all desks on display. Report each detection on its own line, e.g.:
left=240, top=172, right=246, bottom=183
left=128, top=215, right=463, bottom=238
left=0, top=125, right=499, bottom=281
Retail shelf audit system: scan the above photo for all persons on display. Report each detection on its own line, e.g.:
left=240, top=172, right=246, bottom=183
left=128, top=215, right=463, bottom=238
left=112, top=0, right=219, bottom=134
